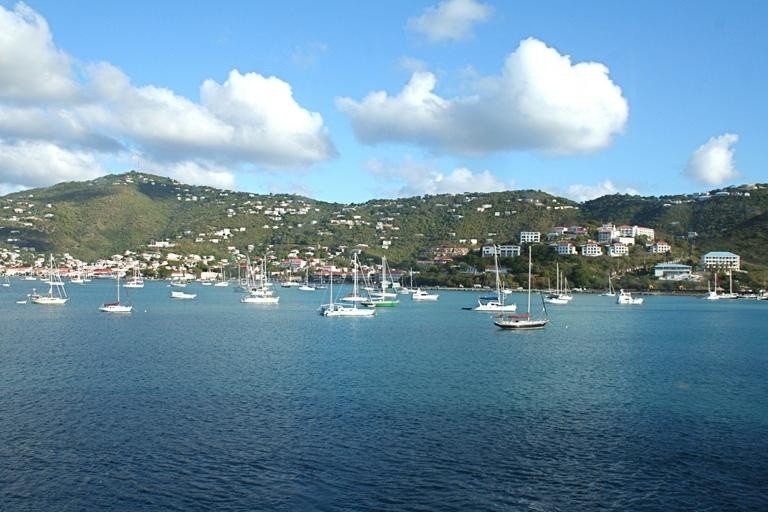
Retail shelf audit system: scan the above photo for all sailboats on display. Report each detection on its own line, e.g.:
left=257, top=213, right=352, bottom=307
left=544, top=261, right=573, bottom=304
left=474, top=246, right=517, bottom=312
left=280, top=252, right=429, bottom=316
left=30, top=253, right=69, bottom=305
left=697, top=267, right=768, bottom=301
left=601, top=275, right=616, bottom=296
left=98, top=263, right=133, bottom=312
left=493, top=246, right=551, bottom=330
left=0, top=261, right=94, bottom=286
left=169, top=254, right=280, bottom=303
left=123, top=262, right=144, bottom=289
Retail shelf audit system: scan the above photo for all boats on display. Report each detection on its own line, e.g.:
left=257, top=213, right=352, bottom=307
left=617, top=289, right=643, bottom=305
left=479, top=287, right=513, bottom=300
left=170, top=291, right=197, bottom=300
left=412, top=287, right=440, bottom=301
